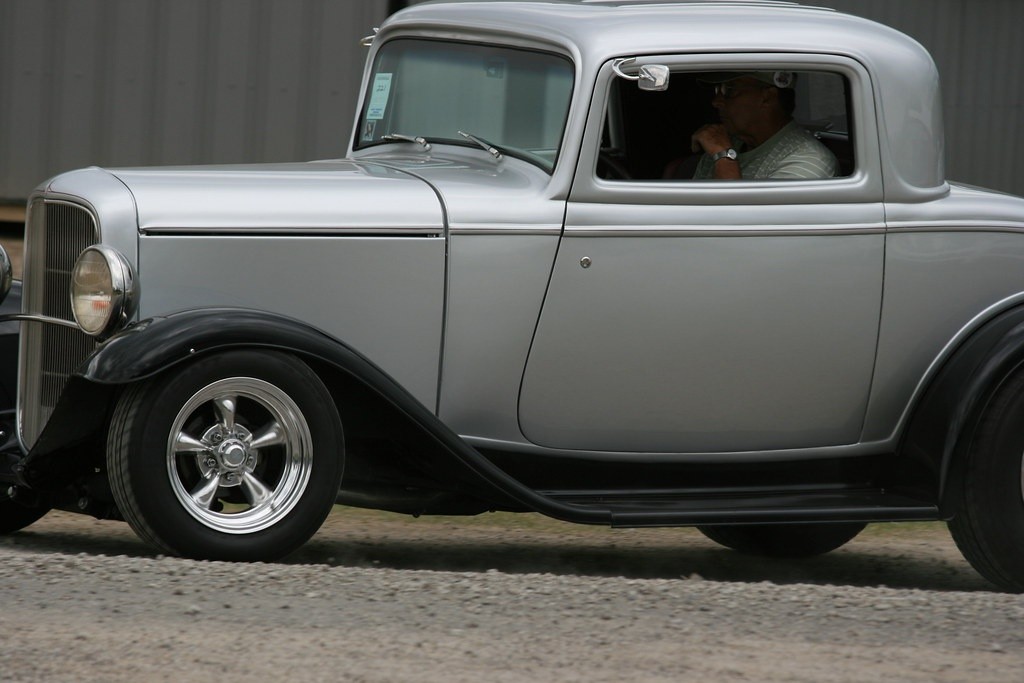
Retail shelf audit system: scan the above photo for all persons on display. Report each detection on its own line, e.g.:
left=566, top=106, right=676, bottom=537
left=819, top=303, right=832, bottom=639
left=687, top=69, right=839, bottom=181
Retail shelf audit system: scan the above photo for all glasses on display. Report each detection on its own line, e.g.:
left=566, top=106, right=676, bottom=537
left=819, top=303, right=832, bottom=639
left=712, top=82, right=764, bottom=99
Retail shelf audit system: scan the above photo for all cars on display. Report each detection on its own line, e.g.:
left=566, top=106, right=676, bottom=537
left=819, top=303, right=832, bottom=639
left=0, top=0, right=1024, bottom=591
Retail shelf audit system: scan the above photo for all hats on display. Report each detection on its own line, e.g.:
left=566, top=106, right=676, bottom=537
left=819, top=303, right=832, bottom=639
left=700, top=72, right=803, bottom=94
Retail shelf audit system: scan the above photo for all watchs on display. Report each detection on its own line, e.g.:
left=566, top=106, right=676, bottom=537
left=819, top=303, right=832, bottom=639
left=711, top=148, right=739, bottom=162
